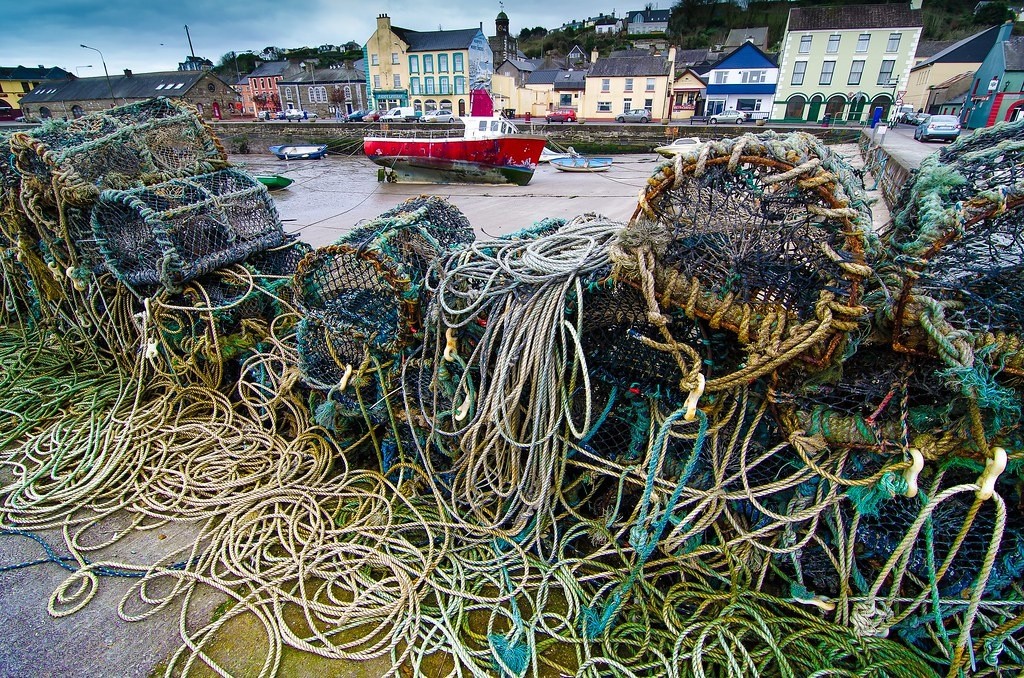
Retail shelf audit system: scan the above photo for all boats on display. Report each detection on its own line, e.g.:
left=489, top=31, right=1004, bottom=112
left=362, top=89, right=546, bottom=188
left=251, top=175, right=295, bottom=190
left=653, top=137, right=705, bottom=159
left=548, top=155, right=614, bottom=173
left=269, top=145, right=328, bottom=160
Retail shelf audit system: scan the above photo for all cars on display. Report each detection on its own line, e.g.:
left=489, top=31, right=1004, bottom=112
left=346, top=107, right=455, bottom=123
left=544, top=109, right=577, bottom=122
left=15, top=116, right=26, bottom=122
left=615, top=109, right=652, bottom=123
left=707, top=109, right=750, bottom=125
left=258, top=109, right=318, bottom=120
left=898, top=103, right=959, bottom=145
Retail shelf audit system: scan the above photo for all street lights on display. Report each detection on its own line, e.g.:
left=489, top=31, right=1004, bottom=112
left=234, top=49, right=254, bottom=114
left=76, top=65, right=93, bottom=77
left=79, top=43, right=116, bottom=107
